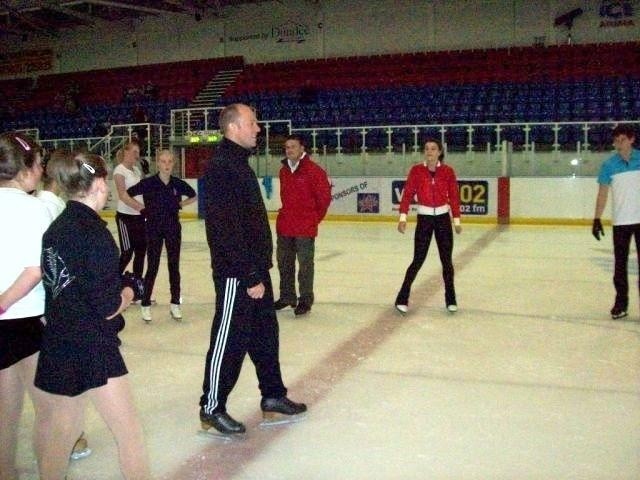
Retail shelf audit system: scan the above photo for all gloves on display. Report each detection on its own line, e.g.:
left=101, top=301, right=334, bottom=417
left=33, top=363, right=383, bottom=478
left=121, top=272, right=146, bottom=302
left=592, top=218, right=604, bottom=240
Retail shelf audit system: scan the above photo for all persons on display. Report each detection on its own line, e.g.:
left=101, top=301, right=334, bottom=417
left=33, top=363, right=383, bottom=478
left=114, top=142, right=157, bottom=304
left=30, top=140, right=68, bottom=220
left=396, top=138, right=463, bottom=313
left=125, top=148, right=199, bottom=323
left=32, top=148, right=150, bottom=479
left=54, top=80, right=159, bottom=136
left=200, top=101, right=308, bottom=434
left=1, top=132, right=88, bottom=479
left=273, top=136, right=333, bottom=315
left=129, top=129, right=147, bottom=147
left=594, top=126, right=639, bottom=315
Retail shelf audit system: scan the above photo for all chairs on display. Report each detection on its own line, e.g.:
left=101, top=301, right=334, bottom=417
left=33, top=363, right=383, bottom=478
left=240, top=51, right=436, bottom=147
left=434, top=40, right=640, bottom=153
left=0, top=67, right=113, bottom=140
left=113, top=55, right=243, bottom=136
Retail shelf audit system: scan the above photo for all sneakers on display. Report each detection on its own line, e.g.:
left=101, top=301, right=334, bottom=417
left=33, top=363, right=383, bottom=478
left=260, top=397, right=309, bottom=417
left=610, top=306, right=624, bottom=316
left=275, top=298, right=297, bottom=311
left=293, top=303, right=309, bottom=315
left=169, top=303, right=183, bottom=320
left=199, top=406, right=245, bottom=436
left=141, top=305, right=153, bottom=322
left=397, top=305, right=408, bottom=313
left=447, top=305, right=458, bottom=311
